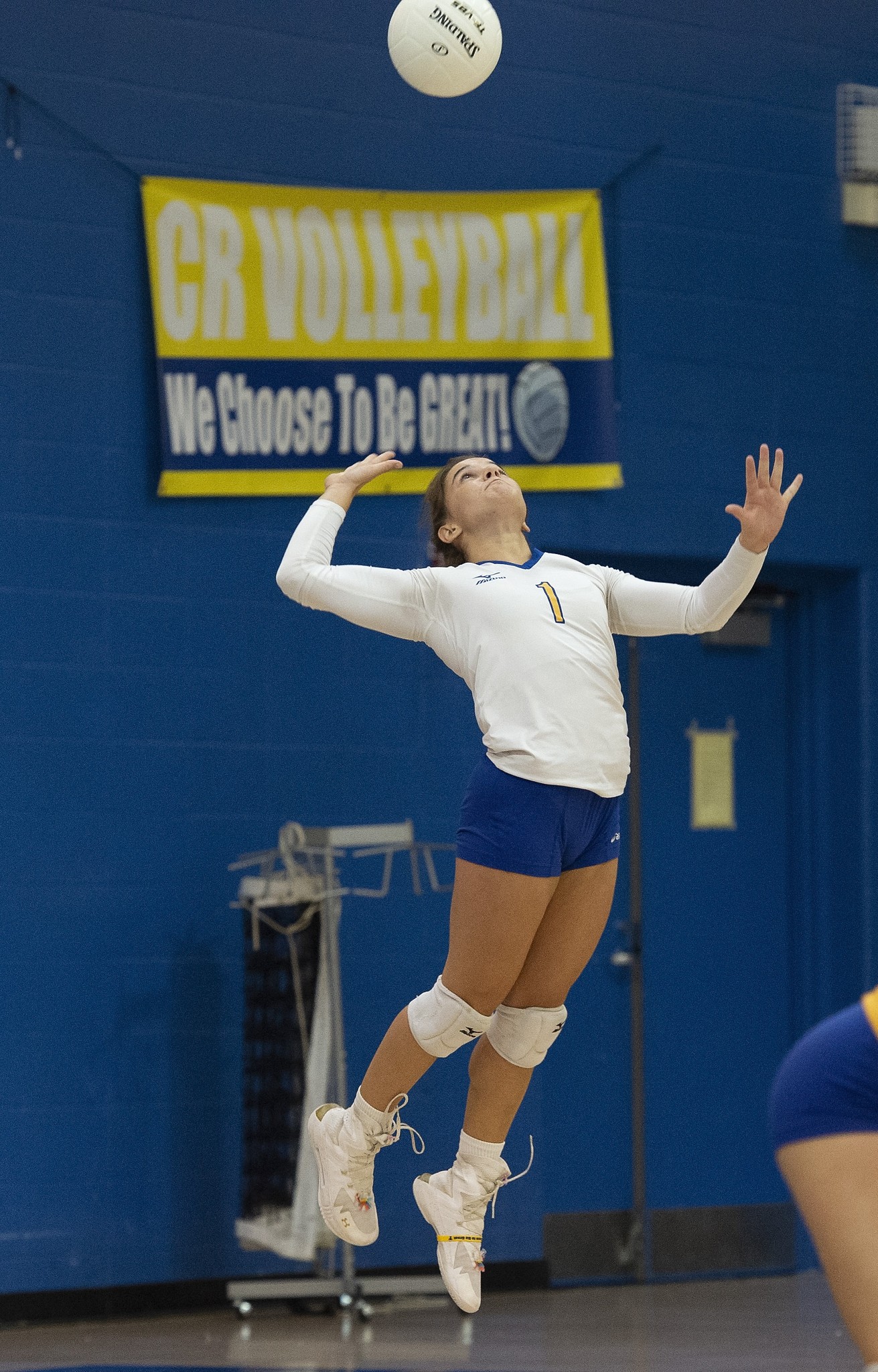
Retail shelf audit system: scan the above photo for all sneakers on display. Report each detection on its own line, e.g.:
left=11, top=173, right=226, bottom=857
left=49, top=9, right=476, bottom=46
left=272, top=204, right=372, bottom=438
left=306, top=1092, right=425, bottom=1248
left=412, top=1135, right=535, bottom=1314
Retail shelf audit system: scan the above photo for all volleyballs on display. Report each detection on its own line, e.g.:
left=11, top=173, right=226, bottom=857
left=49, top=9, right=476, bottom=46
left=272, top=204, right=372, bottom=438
left=387, top=0, right=503, bottom=99
left=510, top=358, right=572, bottom=464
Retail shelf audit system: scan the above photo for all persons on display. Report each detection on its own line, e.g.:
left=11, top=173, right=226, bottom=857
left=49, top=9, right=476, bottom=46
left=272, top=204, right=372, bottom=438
left=277, top=443, right=804, bottom=1314
left=765, top=983, right=878, bottom=1372
